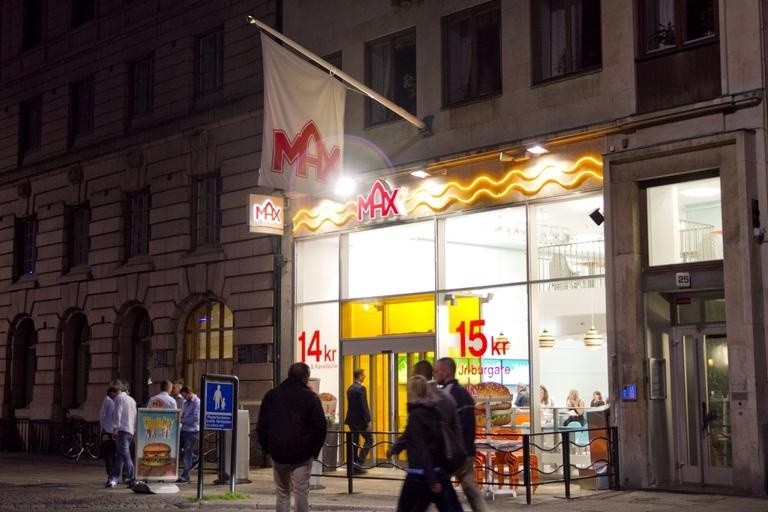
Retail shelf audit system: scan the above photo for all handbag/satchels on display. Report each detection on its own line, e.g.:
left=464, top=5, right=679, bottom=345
left=442, top=421, right=468, bottom=462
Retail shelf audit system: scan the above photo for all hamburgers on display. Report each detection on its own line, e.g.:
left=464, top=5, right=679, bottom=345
left=473, top=382, right=512, bottom=428
left=141, top=443, right=171, bottom=467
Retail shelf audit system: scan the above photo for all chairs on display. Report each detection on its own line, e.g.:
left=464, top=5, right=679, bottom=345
left=455, top=409, right=589, bottom=494
left=543, top=251, right=580, bottom=290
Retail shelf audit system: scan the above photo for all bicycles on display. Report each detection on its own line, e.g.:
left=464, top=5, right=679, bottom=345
left=57, top=411, right=100, bottom=463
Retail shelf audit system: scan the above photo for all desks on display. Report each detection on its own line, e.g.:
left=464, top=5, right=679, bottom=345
left=574, top=256, right=604, bottom=288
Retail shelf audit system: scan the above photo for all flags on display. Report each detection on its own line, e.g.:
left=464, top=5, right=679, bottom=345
left=256, top=29, right=349, bottom=202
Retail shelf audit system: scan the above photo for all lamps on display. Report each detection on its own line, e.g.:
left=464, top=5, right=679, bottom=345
left=583, top=313, right=603, bottom=348
left=494, top=332, right=510, bottom=350
left=538, top=328, right=555, bottom=348
left=498, top=147, right=528, bottom=162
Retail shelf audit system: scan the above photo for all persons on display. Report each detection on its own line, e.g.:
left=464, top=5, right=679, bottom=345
left=512, top=381, right=608, bottom=431
left=96, top=374, right=204, bottom=495
left=432, top=357, right=486, bottom=511
left=341, top=366, right=374, bottom=475
left=387, top=373, right=463, bottom=511
left=250, top=361, right=328, bottom=512
left=413, top=359, right=464, bottom=479
